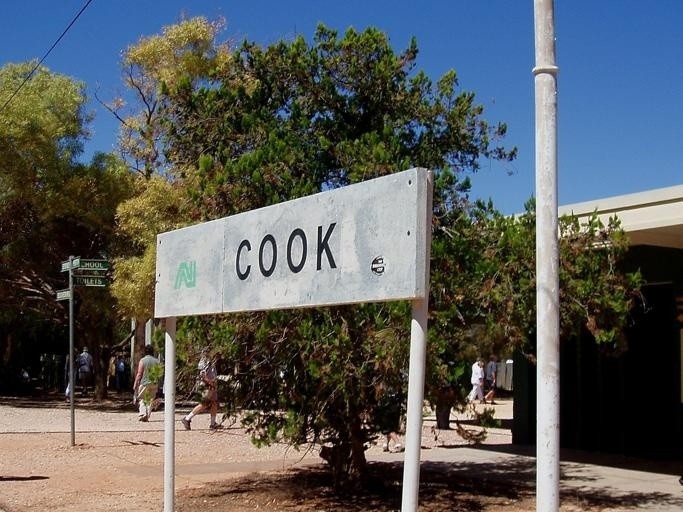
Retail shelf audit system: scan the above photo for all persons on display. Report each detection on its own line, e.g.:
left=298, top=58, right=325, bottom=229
left=484, top=355, right=498, bottom=405
left=131, top=344, right=159, bottom=421
left=464, top=357, right=487, bottom=405
left=179, top=345, right=225, bottom=430
left=62, top=345, right=130, bottom=404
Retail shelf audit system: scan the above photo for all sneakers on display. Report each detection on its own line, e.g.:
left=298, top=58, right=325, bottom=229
left=182, top=416, right=192, bottom=430
left=210, top=422, right=225, bottom=429
